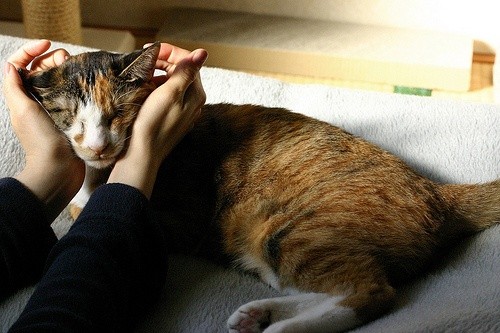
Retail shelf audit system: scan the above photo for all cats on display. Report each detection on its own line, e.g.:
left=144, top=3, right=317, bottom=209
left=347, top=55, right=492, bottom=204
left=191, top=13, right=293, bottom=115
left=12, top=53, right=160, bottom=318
left=16, top=40, right=500, bottom=333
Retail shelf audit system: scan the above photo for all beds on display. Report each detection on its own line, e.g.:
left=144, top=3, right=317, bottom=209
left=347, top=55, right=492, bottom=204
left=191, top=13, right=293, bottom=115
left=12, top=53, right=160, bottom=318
left=1, top=33, right=500, bottom=333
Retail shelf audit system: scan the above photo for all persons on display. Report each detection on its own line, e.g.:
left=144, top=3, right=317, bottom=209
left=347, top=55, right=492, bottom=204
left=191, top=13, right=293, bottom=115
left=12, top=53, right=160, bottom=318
left=0, top=37, right=210, bottom=333
